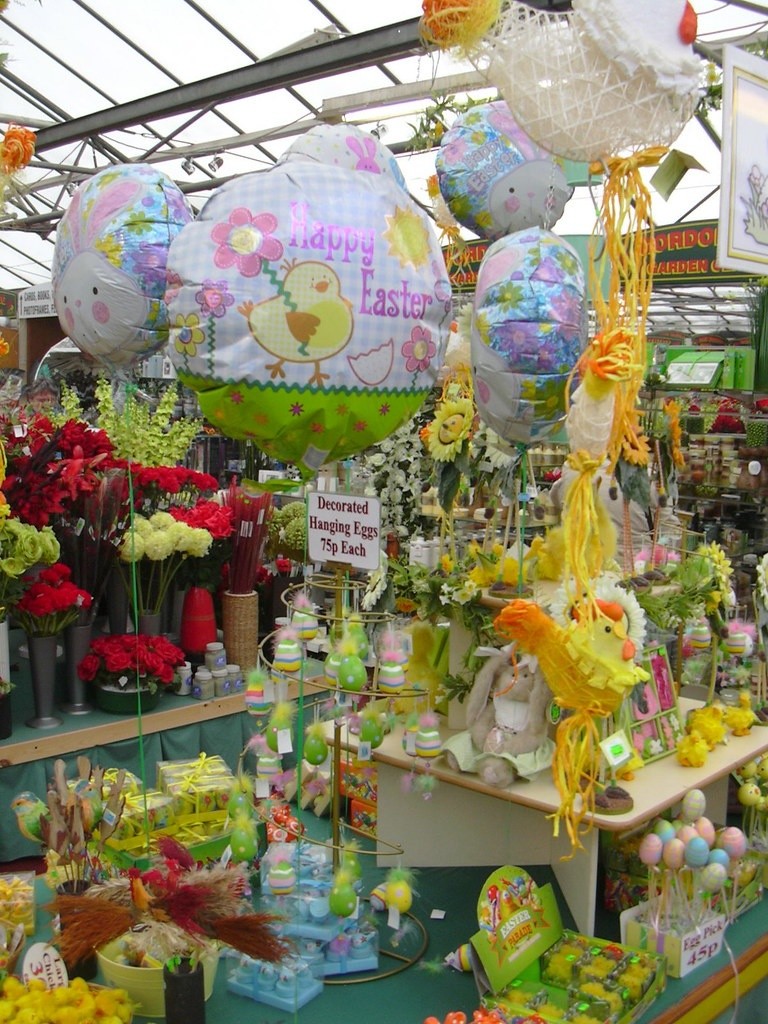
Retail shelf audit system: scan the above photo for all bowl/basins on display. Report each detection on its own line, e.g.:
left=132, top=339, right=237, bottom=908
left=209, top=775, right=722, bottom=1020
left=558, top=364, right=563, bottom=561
left=93, top=936, right=209, bottom=1018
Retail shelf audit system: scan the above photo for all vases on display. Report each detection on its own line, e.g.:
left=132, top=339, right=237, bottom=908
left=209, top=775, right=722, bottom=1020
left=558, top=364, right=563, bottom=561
left=0, top=615, right=15, bottom=740
left=139, top=608, right=164, bottom=635
left=24, top=631, right=64, bottom=732
left=180, top=588, right=217, bottom=669
left=222, top=586, right=262, bottom=669
left=62, top=619, right=94, bottom=717
left=447, top=619, right=476, bottom=731
left=92, top=659, right=164, bottom=718
left=107, top=586, right=127, bottom=636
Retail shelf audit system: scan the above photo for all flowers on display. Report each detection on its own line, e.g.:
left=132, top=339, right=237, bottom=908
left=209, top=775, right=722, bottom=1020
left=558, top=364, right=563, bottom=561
left=361, top=547, right=522, bottom=710
left=0, top=382, right=225, bottom=686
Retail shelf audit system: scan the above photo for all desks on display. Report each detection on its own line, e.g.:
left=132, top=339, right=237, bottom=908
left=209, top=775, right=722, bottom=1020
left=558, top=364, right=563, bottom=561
left=16, top=803, right=768, bottom=1024
left=299, top=693, right=768, bottom=938
left=0, top=654, right=332, bottom=862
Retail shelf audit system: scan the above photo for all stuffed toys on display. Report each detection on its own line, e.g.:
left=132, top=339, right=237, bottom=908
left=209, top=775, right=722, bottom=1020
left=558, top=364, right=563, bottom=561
left=466, top=647, right=549, bottom=758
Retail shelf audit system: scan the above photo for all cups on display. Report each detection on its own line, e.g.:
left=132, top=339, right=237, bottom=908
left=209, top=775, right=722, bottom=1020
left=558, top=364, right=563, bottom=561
left=163, top=956, right=206, bottom=1024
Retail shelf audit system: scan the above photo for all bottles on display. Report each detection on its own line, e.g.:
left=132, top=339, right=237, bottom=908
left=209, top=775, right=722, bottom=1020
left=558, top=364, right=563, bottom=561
left=174, top=642, right=243, bottom=701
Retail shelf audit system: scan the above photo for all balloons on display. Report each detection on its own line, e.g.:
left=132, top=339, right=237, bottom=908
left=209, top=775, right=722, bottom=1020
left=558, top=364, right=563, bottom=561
left=53, top=98, right=589, bottom=466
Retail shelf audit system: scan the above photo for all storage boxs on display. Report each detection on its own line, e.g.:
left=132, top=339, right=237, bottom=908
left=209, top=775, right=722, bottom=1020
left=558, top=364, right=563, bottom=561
left=619, top=890, right=729, bottom=978
left=47, top=753, right=394, bottom=1014
left=614, top=644, right=686, bottom=766
